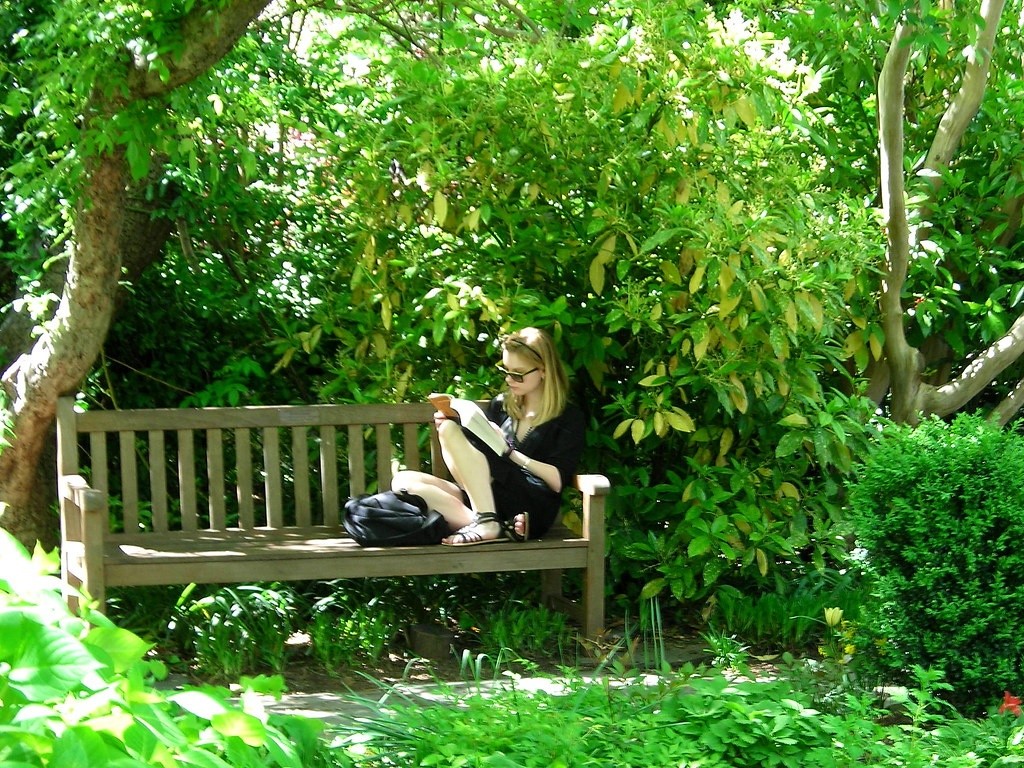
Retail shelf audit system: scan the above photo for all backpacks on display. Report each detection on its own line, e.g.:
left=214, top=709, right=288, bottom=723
left=342, top=490, right=448, bottom=547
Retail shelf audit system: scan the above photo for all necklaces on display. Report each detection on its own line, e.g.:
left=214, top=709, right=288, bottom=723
left=508, top=418, right=534, bottom=451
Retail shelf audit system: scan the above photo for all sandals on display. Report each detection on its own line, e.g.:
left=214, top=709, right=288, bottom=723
left=442, top=512, right=509, bottom=546
left=502, top=512, right=528, bottom=542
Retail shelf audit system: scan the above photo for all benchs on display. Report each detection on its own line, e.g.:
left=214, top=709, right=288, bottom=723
left=57, top=395, right=611, bottom=657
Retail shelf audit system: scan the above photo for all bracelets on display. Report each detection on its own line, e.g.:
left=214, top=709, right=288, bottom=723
left=521, top=454, right=530, bottom=472
left=505, top=442, right=516, bottom=458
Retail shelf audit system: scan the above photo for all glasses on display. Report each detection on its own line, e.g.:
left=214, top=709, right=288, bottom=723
left=495, top=360, right=538, bottom=382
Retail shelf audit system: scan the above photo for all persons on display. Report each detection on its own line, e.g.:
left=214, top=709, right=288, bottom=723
left=391, top=326, right=585, bottom=547
